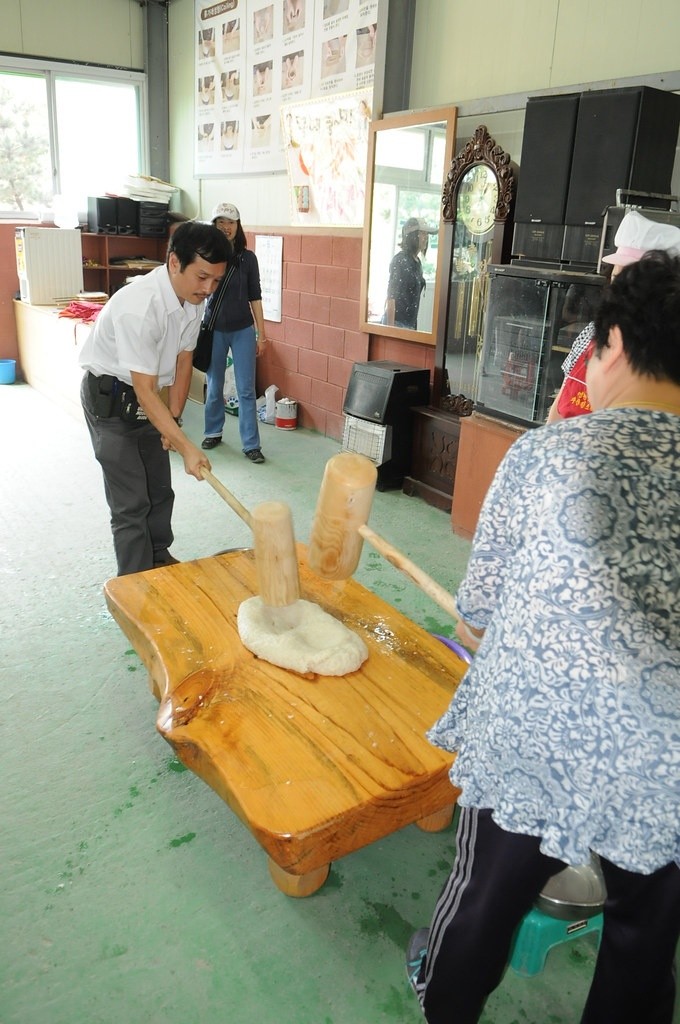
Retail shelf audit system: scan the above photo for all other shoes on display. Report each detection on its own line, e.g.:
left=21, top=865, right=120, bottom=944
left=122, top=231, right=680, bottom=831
left=245, top=449, right=265, bottom=462
left=202, top=436, right=222, bottom=449
left=156, top=558, right=179, bottom=566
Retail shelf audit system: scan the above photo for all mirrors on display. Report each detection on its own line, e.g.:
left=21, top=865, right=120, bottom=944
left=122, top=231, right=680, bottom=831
left=358, top=105, right=459, bottom=346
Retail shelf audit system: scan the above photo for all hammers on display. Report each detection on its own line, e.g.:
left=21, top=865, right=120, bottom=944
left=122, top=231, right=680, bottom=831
left=198, top=466, right=303, bottom=606
left=307, top=450, right=458, bottom=620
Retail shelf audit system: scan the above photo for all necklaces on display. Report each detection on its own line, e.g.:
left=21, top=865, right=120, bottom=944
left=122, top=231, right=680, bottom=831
left=608, top=403, right=680, bottom=409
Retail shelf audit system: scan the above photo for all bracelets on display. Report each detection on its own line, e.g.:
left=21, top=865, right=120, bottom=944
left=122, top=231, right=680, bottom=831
left=174, top=417, right=184, bottom=427
left=257, top=338, right=267, bottom=343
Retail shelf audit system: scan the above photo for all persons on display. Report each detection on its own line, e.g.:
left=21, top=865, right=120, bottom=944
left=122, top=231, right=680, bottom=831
left=78, top=220, right=233, bottom=576
left=544, top=209, right=680, bottom=423
left=202, top=202, right=268, bottom=463
left=405, top=244, right=680, bottom=1024
left=382, top=217, right=439, bottom=331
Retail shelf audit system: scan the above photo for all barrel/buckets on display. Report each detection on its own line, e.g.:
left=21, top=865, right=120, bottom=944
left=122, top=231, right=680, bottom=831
left=276, top=402, right=297, bottom=431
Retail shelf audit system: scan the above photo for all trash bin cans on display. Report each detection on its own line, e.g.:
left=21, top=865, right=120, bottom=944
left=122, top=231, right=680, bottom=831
left=0, top=359, right=16, bottom=385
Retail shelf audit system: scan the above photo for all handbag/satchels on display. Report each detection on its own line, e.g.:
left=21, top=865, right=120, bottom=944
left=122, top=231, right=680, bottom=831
left=120, top=386, right=150, bottom=423
left=193, top=330, right=212, bottom=372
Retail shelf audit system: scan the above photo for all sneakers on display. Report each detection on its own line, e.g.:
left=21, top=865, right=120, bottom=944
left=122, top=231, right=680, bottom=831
left=406, top=928, right=433, bottom=1024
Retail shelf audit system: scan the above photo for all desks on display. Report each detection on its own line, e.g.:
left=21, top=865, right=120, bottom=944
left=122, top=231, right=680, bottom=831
left=103, top=538, right=471, bottom=898
left=451, top=410, right=531, bottom=540
left=13, top=298, right=97, bottom=424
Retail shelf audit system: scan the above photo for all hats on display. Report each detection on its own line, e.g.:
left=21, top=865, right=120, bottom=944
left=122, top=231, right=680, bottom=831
left=212, top=204, right=240, bottom=223
left=602, top=211, right=680, bottom=266
left=403, top=218, right=438, bottom=235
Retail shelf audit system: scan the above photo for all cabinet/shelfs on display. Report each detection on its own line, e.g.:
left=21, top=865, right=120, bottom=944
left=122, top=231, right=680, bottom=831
left=81, top=233, right=168, bottom=300
left=473, top=264, right=607, bottom=430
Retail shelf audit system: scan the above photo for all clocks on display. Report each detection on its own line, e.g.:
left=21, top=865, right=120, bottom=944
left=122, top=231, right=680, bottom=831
left=402, top=125, right=516, bottom=515
